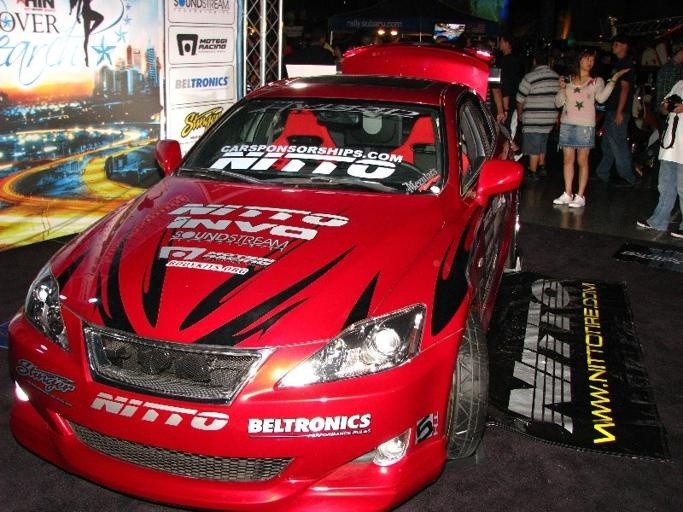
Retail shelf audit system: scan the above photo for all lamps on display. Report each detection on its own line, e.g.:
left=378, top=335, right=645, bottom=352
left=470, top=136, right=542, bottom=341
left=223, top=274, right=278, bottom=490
left=390, top=23, right=399, bottom=37
left=377, top=22, right=386, bottom=35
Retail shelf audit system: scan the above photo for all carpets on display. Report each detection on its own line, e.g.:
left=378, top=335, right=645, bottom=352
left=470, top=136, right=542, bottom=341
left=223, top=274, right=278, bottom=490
left=0, top=221, right=682, bottom=511
left=484, top=272, right=675, bottom=465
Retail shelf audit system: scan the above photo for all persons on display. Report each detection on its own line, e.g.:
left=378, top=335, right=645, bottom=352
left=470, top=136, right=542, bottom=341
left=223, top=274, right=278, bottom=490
left=247, top=23, right=496, bottom=94
left=490, top=24, right=682, bottom=239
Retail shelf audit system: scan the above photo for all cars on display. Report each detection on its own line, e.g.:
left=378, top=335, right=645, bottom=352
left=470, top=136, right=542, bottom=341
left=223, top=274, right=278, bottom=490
left=6, top=41, right=523, bottom=512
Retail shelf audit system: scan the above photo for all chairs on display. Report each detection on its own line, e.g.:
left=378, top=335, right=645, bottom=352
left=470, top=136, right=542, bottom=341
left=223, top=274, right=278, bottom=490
left=268, top=109, right=336, bottom=172
left=387, top=116, right=470, bottom=186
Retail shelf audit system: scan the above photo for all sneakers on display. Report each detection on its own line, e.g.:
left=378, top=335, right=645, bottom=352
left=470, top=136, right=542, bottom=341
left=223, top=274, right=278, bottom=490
left=524, top=168, right=540, bottom=182
left=538, top=165, right=547, bottom=177
left=554, top=192, right=573, bottom=204
left=636, top=218, right=652, bottom=229
left=671, top=230, right=683, bottom=238
left=569, top=194, right=586, bottom=208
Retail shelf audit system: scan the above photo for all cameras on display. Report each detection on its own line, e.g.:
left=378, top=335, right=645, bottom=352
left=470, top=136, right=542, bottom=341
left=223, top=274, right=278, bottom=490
left=564, top=76, right=571, bottom=83
left=666, top=94, right=681, bottom=112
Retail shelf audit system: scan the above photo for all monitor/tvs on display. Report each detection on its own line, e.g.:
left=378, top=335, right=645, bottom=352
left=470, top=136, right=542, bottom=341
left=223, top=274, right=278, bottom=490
left=432, top=21, right=466, bottom=43
